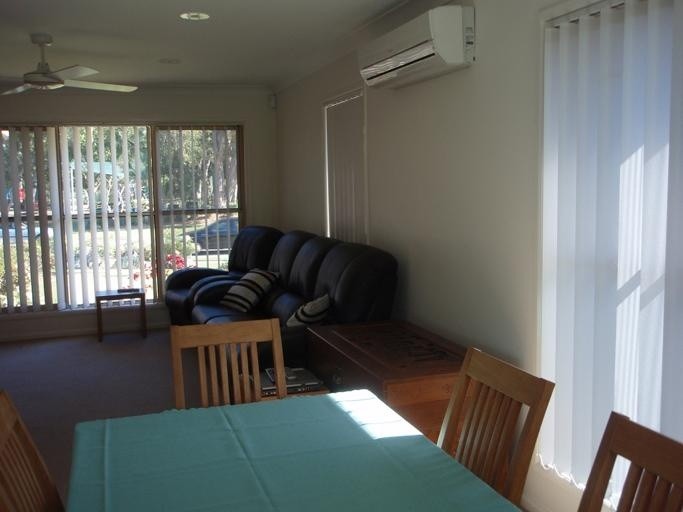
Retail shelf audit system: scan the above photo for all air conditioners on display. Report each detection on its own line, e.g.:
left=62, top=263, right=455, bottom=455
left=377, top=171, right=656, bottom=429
left=358, top=5, right=477, bottom=88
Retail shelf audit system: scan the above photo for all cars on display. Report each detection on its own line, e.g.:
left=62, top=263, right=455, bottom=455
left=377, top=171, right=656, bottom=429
left=176, top=216, right=239, bottom=252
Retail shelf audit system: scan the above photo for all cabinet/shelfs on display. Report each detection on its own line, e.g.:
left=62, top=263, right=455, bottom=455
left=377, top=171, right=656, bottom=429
left=307, top=321, right=522, bottom=473
left=235, top=372, right=330, bottom=401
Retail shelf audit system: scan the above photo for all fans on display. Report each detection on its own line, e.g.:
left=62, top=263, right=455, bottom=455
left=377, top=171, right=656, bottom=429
left=0, top=33, right=138, bottom=96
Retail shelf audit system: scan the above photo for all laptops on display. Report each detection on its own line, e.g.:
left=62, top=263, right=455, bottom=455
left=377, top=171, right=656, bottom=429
left=250, top=367, right=319, bottom=395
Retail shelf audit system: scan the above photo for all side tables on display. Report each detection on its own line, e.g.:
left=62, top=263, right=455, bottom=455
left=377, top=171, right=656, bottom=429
left=95, top=288, right=148, bottom=342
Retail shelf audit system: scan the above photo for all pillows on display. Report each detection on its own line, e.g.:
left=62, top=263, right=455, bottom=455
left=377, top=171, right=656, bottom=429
left=286, top=292, right=331, bottom=327
left=218, top=266, right=281, bottom=312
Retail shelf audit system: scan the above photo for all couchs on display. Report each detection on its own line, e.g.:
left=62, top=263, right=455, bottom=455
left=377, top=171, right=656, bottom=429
left=191, top=231, right=401, bottom=389
left=166, top=225, right=284, bottom=327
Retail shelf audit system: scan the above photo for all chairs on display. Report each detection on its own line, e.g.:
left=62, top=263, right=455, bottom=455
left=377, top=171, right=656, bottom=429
left=0, top=390, right=64, bottom=512
left=168, top=318, right=288, bottom=408
left=436, top=345, right=557, bottom=505
left=577, top=411, right=683, bottom=512
